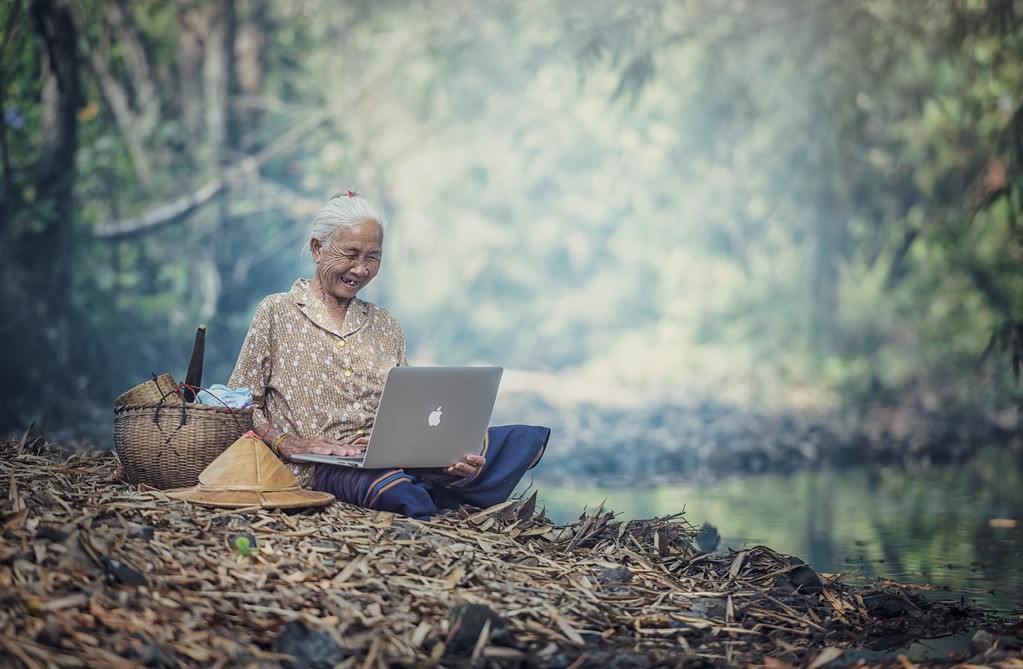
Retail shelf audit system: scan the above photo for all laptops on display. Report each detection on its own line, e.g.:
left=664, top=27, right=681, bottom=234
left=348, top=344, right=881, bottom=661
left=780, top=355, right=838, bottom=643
left=290, top=366, right=504, bottom=468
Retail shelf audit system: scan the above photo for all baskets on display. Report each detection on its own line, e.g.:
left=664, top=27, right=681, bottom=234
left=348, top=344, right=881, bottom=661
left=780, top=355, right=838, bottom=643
left=114, top=402, right=253, bottom=488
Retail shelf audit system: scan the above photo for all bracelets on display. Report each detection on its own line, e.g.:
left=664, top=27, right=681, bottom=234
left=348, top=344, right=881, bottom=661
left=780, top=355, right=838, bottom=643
left=270, top=434, right=292, bottom=456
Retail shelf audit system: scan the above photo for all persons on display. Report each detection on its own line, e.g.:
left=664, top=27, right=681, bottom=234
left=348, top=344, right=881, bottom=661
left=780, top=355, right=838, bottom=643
left=226, top=188, right=552, bottom=520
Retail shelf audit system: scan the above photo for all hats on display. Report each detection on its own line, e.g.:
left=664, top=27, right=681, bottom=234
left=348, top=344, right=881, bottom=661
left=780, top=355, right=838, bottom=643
left=164, top=430, right=335, bottom=508
